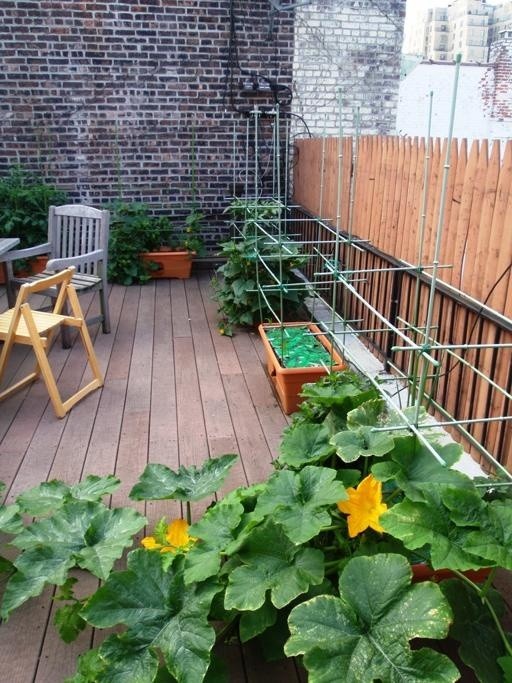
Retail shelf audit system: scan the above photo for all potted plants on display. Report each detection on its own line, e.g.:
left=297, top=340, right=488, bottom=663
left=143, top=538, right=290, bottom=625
left=0, top=161, right=67, bottom=286
left=101, top=200, right=208, bottom=279
left=258, top=318, right=349, bottom=416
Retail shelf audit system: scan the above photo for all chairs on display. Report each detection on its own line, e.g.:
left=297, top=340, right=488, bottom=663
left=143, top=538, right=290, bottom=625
left=0, top=203, right=113, bottom=420
